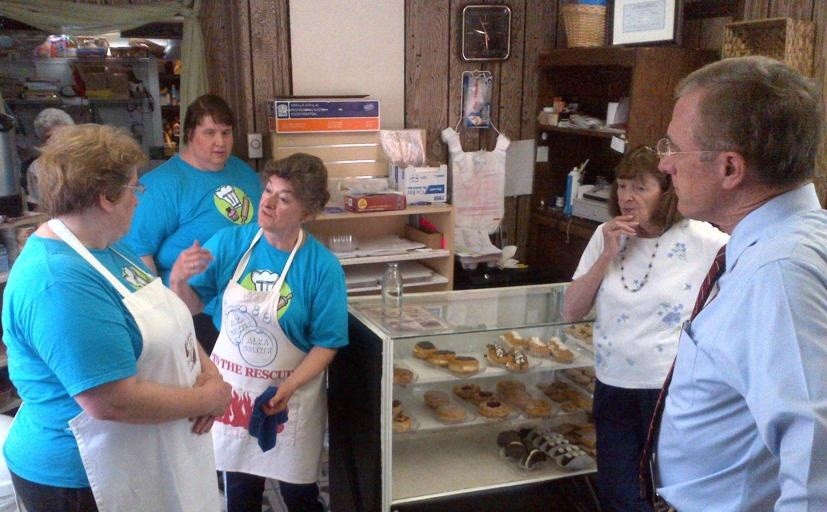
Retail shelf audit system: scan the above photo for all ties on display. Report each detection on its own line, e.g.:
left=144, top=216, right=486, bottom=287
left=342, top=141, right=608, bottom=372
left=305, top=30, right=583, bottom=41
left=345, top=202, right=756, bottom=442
left=635, top=243, right=732, bottom=504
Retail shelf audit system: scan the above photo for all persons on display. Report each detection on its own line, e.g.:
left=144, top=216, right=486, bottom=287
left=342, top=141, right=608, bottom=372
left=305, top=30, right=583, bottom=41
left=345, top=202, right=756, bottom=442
left=637, top=53, right=826, bottom=511
left=27, top=107, right=77, bottom=214
left=0, top=121, right=233, bottom=512
left=166, top=151, right=347, bottom=512
left=559, top=144, right=726, bottom=512
left=116, top=89, right=263, bottom=359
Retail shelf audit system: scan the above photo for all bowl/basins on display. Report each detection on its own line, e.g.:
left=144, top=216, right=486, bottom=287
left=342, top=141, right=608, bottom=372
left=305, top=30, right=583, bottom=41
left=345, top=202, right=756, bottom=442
left=325, top=234, right=361, bottom=252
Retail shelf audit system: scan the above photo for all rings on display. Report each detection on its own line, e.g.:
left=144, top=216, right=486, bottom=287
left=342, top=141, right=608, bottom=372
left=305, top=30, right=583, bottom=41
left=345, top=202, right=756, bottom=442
left=190, top=262, right=194, bottom=269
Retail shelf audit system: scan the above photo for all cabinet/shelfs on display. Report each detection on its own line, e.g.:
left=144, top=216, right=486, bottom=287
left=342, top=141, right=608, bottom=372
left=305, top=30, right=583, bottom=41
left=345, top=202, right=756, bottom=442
left=326, top=281, right=602, bottom=512
left=525, top=45, right=724, bottom=278
left=302, top=198, right=455, bottom=296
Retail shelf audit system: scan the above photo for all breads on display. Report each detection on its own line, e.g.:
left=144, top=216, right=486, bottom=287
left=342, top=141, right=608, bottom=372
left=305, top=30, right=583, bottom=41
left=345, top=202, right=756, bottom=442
left=449, top=355, right=478, bottom=374
left=484, top=324, right=594, bottom=372
left=392, top=400, right=411, bottom=431
left=424, top=370, right=596, bottom=474
left=428, top=349, right=455, bottom=366
left=414, top=342, right=436, bottom=359
left=393, top=369, right=414, bottom=385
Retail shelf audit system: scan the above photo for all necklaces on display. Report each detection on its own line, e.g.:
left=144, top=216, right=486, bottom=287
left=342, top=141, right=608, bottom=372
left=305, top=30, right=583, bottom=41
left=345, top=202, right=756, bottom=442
left=619, top=229, right=661, bottom=293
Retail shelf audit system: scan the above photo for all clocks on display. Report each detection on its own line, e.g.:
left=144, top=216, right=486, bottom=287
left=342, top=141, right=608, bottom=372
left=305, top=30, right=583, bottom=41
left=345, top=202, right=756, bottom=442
left=460, top=4, right=513, bottom=63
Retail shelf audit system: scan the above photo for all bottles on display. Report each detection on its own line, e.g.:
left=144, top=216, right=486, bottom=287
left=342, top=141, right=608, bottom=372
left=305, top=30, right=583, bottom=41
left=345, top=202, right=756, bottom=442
left=170, top=84, right=177, bottom=106
left=50, top=41, right=58, bottom=57
left=565, top=167, right=581, bottom=216
left=381, top=263, right=403, bottom=319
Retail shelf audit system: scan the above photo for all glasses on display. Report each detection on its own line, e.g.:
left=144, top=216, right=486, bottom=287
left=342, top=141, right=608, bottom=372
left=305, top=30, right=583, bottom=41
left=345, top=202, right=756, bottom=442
left=123, top=181, right=145, bottom=197
left=654, top=136, right=718, bottom=162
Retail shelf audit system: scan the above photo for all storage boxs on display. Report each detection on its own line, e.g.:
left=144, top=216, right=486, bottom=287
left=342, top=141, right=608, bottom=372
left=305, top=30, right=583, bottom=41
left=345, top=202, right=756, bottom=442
left=266, top=93, right=381, bottom=134
left=31, top=57, right=76, bottom=89
left=387, top=158, right=449, bottom=205
